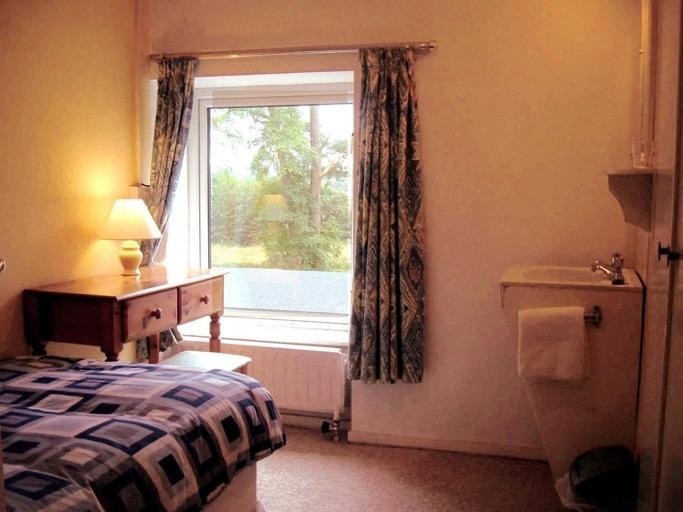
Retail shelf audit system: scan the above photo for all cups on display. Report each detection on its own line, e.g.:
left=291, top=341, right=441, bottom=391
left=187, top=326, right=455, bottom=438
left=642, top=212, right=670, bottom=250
left=631, top=140, right=652, bottom=169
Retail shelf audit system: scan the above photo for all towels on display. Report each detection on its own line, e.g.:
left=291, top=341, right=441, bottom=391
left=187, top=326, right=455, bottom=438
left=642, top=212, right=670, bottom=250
left=515, top=304, right=593, bottom=383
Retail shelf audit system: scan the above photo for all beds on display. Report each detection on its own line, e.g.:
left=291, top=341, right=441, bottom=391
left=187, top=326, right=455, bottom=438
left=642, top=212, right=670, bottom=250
left=0, top=349, right=288, bottom=512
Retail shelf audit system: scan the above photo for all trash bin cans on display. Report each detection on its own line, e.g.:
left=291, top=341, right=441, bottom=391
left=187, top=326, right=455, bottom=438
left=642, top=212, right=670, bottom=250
left=554, top=444, right=639, bottom=512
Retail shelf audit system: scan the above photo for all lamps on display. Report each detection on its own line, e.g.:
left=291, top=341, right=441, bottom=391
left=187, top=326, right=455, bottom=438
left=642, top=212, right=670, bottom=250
left=100, top=197, right=164, bottom=277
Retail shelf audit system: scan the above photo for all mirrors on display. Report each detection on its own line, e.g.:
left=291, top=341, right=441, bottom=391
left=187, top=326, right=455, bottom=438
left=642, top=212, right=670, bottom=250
left=636, top=0, right=655, bottom=162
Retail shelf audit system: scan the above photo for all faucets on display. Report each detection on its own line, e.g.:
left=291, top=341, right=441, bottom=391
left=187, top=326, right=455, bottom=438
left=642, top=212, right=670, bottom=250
left=590, top=252, right=625, bottom=282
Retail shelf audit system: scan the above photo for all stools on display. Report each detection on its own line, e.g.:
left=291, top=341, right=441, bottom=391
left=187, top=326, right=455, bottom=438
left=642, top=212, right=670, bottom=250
left=157, top=348, right=252, bottom=378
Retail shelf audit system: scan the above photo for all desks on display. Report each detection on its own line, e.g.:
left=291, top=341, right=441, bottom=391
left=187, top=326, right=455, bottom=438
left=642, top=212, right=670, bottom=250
left=23, top=264, right=230, bottom=366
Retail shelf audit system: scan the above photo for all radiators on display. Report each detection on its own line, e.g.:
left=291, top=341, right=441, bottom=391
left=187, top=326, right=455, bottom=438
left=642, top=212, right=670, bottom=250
left=162, top=337, right=348, bottom=445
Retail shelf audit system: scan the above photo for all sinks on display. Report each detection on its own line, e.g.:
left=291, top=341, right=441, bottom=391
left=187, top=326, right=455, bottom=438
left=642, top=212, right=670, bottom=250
left=522, top=266, right=597, bottom=282
